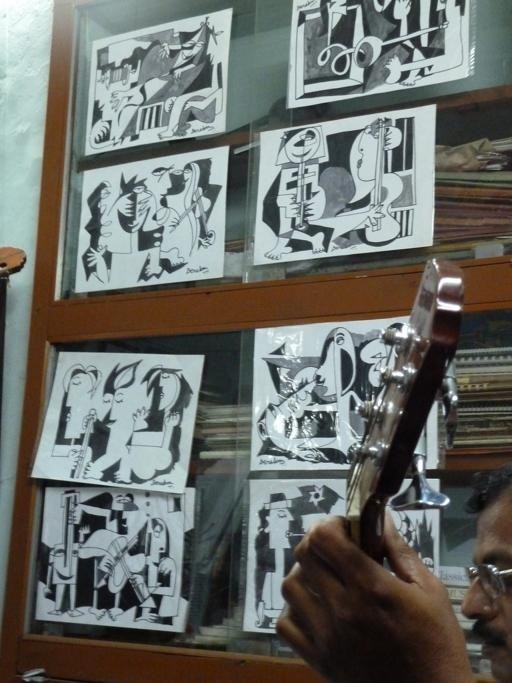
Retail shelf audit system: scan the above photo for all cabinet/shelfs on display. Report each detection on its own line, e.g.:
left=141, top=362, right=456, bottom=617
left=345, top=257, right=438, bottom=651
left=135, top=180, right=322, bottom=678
left=0, top=0, right=511, bottom=682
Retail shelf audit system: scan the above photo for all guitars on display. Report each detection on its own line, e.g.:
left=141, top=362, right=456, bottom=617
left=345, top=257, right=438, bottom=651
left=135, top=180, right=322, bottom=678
left=340, top=256, right=467, bottom=568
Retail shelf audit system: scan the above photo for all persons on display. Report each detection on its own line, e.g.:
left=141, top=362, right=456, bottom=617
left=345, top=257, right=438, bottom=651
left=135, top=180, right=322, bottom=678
left=273, top=461, right=511, bottom=683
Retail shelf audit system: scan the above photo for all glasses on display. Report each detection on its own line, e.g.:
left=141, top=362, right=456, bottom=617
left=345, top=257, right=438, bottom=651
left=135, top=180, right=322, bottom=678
left=463, top=562, right=512, bottom=599
